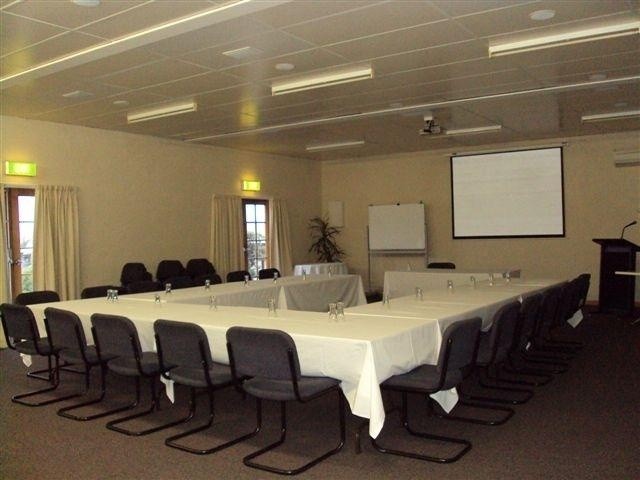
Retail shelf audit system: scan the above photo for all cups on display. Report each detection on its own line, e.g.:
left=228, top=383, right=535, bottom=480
left=336, top=302, right=344, bottom=319
left=267, top=298, right=277, bottom=317
left=209, top=295, right=217, bottom=311
left=488, top=272, right=493, bottom=285
left=244, top=275, right=249, bottom=285
left=447, top=279, right=454, bottom=294
left=329, top=303, right=337, bottom=320
left=416, top=287, right=423, bottom=301
left=383, top=295, right=390, bottom=307
left=107, top=290, right=118, bottom=303
left=327, top=265, right=333, bottom=277
left=274, top=273, right=278, bottom=282
left=205, top=280, right=210, bottom=290
left=166, top=284, right=171, bottom=294
left=506, top=274, right=511, bottom=285
left=302, top=269, right=306, bottom=280
left=155, top=294, right=160, bottom=306
left=469, top=276, right=477, bottom=288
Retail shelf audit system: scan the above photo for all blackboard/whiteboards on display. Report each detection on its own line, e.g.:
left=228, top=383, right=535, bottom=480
left=369, top=203, right=426, bottom=250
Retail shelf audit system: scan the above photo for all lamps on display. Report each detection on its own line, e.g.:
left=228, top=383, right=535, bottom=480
left=581, top=110, right=640, bottom=123
left=446, top=124, right=502, bottom=137
left=127, top=100, right=198, bottom=124
left=306, top=140, right=365, bottom=153
left=489, top=17, right=640, bottom=59
left=271, top=67, right=375, bottom=97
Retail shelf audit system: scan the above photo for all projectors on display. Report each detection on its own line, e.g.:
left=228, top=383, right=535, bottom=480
left=419, top=124, right=444, bottom=136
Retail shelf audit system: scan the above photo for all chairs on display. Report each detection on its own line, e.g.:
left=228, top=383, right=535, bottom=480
left=88, top=313, right=161, bottom=436
left=120, top=263, right=153, bottom=283
left=466, top=293, right=556, bottom=404
left=427, top=263, right=456, bottom=270
left=227, top=271, right=252, bottom=282
left=430, top=301, right=522, bottom=426
left=128, top=282, right=162, bottom=295
left=227, top=324, right=345, bottom=475
left=259, top=268, right=281, bottom=279
left=154, top=320, right=233, bottom=456
left=532, top=273, right=592, bottom=373
left=0, top=303, right=72, bottom=406
left=14, top=291, right=86, bottom=381
left=43, top=307, right=106, bottom=422
left=185, top=259, right=217, bottom=274
left=156, top=260, right=185, bottom=279
left=163, top=277, right=193, bottom=290
left=194, top=274, right=222, bottom=287
left=369, top=317, right=483, bottom=464
left=81, top=285, right=125, bottom=299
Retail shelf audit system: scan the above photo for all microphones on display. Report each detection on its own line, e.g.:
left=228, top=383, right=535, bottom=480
left=620, top=221, right=637, bottom=239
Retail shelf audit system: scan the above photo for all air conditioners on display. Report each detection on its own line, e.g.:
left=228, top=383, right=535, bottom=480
left=614, top=147, right=640, bottom=167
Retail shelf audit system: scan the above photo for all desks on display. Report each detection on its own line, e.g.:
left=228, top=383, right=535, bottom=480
left=21, top=296, right=459, bottom=454
left=329, top=278, right=583, bottom=333
left=294, top=262, right=348, bottom=276
left=118, top=274, right=367, bottom=311
left=383, top=269, right=521, bottom=301
